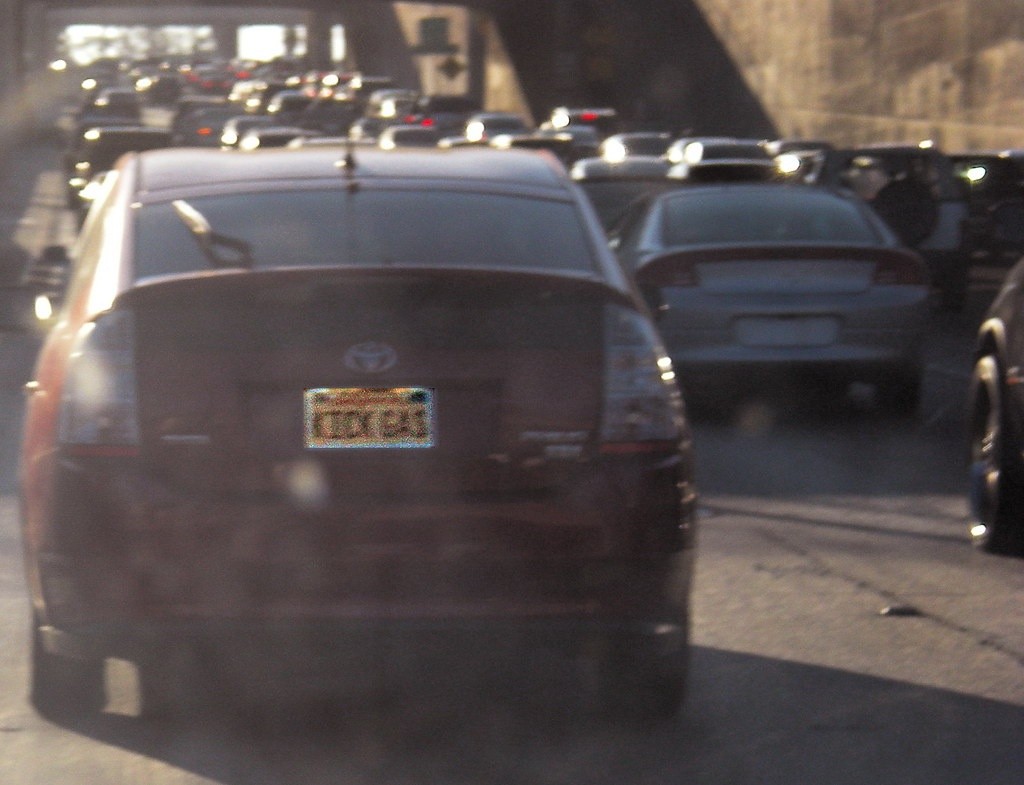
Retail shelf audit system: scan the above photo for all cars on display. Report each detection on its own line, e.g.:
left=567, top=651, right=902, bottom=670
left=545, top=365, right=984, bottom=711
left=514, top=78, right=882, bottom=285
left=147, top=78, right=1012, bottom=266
left=13, top=144, right=707, bottom=731
left=607, top=181, right=934, bottom=406
left=959, top=261, right=1023, bottom=554
left=64, top=51, right=1024, bottom=296
